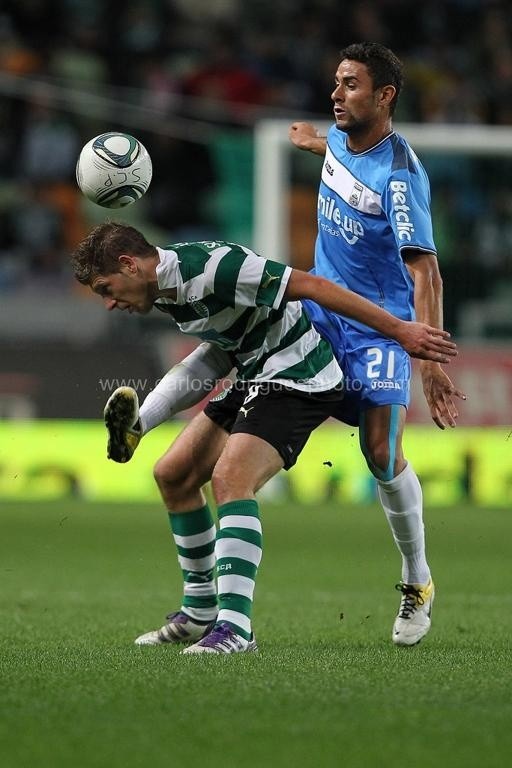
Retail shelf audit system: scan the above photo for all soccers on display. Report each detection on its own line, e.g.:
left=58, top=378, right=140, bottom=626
left=76, top=131, right=153, bottom=207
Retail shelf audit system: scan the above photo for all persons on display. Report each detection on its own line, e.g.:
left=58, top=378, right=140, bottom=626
left=103, top=35, right=469, bottom=646
left=71, top=221, right=462, bottom=655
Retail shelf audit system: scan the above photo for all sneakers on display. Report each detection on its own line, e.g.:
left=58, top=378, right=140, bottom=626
left=136, top=607, right=219, bottom=646
left=391, top=571, right=438, bottom=650
left=182, top=619, right=261, bottom=656
left=104, top=381, right=144, bottom=465
